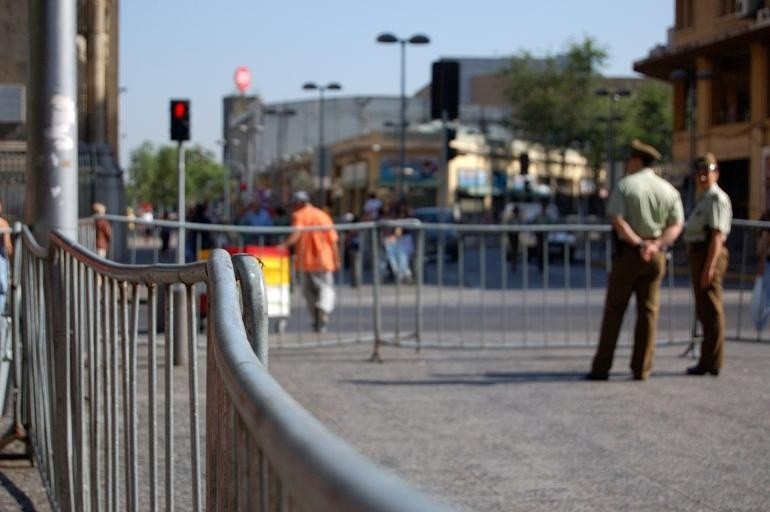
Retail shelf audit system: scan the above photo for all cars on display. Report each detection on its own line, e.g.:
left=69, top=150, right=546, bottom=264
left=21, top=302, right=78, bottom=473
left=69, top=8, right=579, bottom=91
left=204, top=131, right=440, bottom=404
left=348, top=217, right=413, bottom=265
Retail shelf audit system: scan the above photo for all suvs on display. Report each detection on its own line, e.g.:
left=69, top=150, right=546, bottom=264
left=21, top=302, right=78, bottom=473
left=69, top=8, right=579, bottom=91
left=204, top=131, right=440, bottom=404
left=410, top=207, right=458, bottom=261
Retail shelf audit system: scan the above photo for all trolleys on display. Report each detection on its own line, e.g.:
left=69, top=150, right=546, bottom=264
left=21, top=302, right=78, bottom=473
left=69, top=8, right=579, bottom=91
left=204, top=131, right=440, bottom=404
left=184, top=241, right=297, bottom=334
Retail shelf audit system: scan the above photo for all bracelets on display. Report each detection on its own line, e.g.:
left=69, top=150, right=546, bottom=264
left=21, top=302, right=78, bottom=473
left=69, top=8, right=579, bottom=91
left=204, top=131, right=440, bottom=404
left=634, top=239, right=647, bottom=255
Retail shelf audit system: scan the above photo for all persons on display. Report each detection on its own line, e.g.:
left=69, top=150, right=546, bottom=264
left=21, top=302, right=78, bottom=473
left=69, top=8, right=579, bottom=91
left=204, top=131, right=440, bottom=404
left=91, top=202, right=112, bottom=259
left=531, top=204, right=553, bottom=271
left=683, top=153, right=733, bottom=375
left=0, top=216, right=14, bottom=315
left=750, top=212, right=770, bottom=337
left=506, top=207, right=526, bottom=272
left=585, top=139, right=685, bottom=380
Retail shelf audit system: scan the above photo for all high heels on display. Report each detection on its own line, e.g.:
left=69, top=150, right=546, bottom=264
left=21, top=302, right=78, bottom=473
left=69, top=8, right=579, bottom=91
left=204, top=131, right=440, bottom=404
left=689, top=364, right=718, bottom=375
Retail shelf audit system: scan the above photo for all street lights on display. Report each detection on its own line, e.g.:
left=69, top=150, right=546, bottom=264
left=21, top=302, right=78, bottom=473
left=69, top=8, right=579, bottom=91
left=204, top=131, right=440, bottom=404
left=265, top=105, right=297, bottom=202
left=597, top=113, right=625, bottom=193
left=669, top=44, right=714, bottom=215
left=376, top=30, right=433, bottom=207
left=239, top=122, right=263, bottom=203
left=302, top=82, right=341, bottom=208
left=595, top=86, right=632, bottom=206
left=216, top=136, right=241, bottom=220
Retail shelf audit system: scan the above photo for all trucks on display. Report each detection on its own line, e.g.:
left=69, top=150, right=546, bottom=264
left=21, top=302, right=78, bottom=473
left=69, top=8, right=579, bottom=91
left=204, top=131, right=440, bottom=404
left=499, top=200, right=581, bottom=263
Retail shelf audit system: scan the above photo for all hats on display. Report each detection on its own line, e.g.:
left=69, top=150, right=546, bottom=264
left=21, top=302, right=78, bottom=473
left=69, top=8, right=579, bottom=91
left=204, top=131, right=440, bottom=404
left=694, top=153, right=716, bottom=172
left=625, top=139, right=657, bottom=164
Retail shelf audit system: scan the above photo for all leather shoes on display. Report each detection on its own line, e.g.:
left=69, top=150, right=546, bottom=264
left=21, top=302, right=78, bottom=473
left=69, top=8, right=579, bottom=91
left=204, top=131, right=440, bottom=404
left=584, top=371, right=608, bottom=379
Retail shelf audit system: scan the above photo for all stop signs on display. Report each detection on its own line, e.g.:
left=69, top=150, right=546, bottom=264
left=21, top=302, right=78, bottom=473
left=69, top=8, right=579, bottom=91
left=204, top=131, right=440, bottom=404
left=237, top=67, right=251, bottom=91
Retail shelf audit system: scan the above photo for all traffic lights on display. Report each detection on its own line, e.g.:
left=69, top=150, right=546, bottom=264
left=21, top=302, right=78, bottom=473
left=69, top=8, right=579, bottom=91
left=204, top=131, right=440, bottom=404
left=171, top=101, right=191, bottom=141
left=448, top=129, right=458, bottom=160
